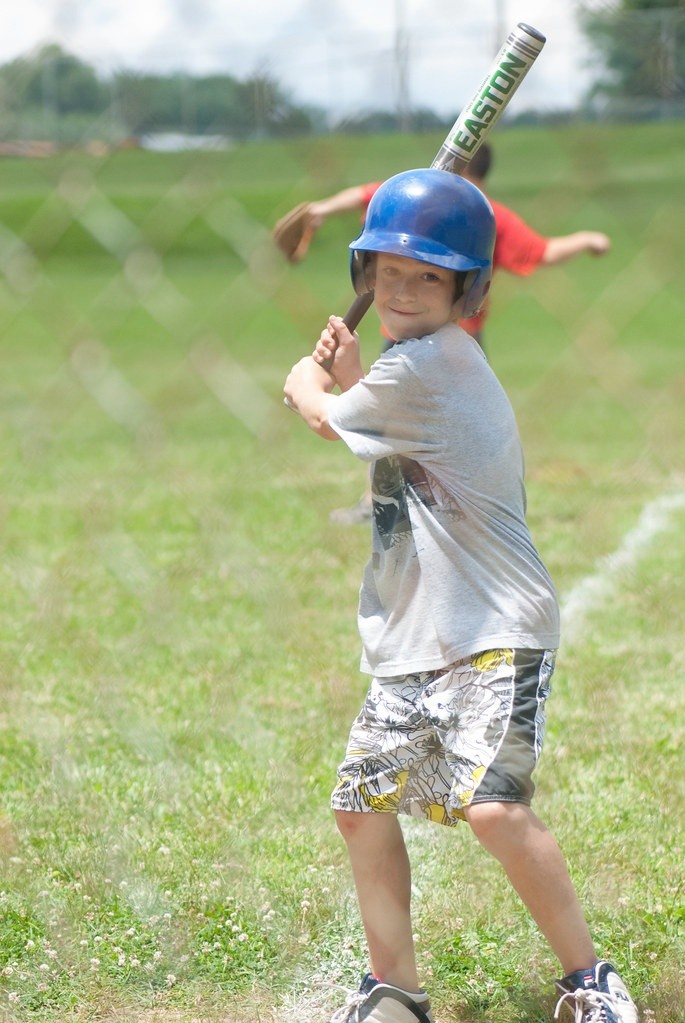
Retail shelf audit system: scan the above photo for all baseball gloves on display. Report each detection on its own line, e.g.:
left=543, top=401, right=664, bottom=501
left=274, top=200, right=317, bottom=267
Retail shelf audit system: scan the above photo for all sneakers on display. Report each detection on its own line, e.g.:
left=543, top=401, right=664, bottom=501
left=314, top=972, right=432, bottom=1023
left=553, top=962, right=638, bottom=1023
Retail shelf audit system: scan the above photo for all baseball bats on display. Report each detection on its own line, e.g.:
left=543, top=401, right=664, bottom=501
left=281, top=21, right=547, bottom=417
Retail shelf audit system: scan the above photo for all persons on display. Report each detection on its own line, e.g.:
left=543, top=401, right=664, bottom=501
left=282, top=169, right=639, bottom=1023
left=274, top=139, right=610, bottom=524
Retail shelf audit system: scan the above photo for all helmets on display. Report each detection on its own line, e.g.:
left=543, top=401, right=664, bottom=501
left=348, top=168, right=496, bottom=319
